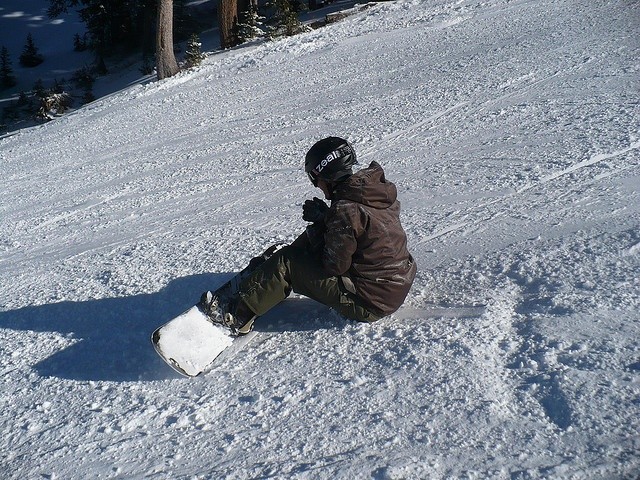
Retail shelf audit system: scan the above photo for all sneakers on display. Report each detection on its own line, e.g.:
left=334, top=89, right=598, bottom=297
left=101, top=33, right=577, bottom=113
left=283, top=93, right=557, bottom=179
left=251, top=257, right=267, bottom=272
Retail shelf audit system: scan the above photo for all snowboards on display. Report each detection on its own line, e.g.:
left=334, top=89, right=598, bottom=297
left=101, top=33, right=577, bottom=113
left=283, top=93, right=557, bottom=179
left=150, top=242, right=293, bottom=378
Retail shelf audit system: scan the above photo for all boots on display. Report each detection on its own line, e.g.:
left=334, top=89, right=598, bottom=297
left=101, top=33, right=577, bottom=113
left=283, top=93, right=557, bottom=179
left=200, top=290, right=259, bottom=336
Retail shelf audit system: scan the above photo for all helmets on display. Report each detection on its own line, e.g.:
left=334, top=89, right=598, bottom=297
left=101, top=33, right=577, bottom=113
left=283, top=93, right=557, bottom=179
left=305, top=137, right=359, bottom=180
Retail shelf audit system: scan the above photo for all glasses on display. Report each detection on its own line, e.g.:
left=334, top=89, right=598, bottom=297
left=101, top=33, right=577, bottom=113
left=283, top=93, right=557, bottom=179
left=307, top=145, right=353, bottom=187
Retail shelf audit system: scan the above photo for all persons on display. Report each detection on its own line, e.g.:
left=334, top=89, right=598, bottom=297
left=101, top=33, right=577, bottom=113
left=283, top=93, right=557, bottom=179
left=201, top=137, right=418, bottom=336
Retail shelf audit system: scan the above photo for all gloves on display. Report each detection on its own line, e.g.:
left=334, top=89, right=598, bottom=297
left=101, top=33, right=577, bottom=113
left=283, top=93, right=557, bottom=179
left=302, top=197, right=331, bottom=222
